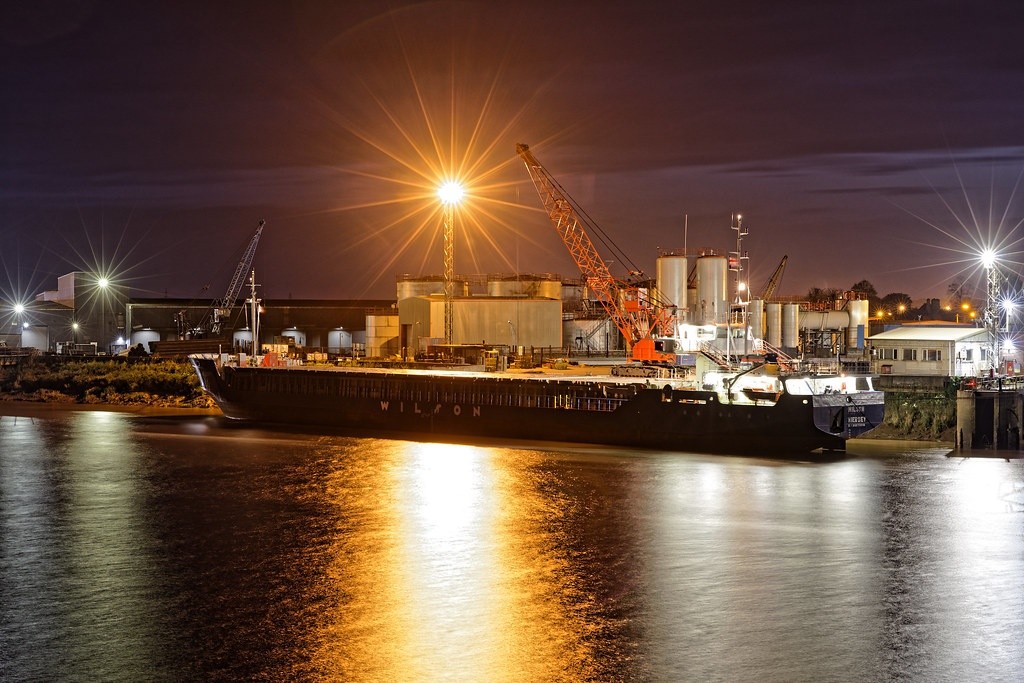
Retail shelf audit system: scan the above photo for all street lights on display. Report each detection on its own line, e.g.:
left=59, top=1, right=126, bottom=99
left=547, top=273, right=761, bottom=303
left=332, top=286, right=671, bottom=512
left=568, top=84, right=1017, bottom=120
left=977, top=245, right=1019, bottom=369
left=434, top=177, right=472, bottom=346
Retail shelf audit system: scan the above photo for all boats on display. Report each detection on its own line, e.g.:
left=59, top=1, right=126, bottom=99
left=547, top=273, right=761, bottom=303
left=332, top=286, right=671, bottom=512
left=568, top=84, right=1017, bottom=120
left=188, top=354, right=885, bottom=456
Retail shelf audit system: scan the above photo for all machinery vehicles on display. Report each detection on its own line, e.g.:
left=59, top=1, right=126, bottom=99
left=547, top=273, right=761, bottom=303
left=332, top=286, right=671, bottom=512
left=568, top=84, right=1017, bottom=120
left=728, top=255, right=789, bottom=340
left=176, top=219, right=265, bottom=339
left=515, top=142, right=688, bottom=377
